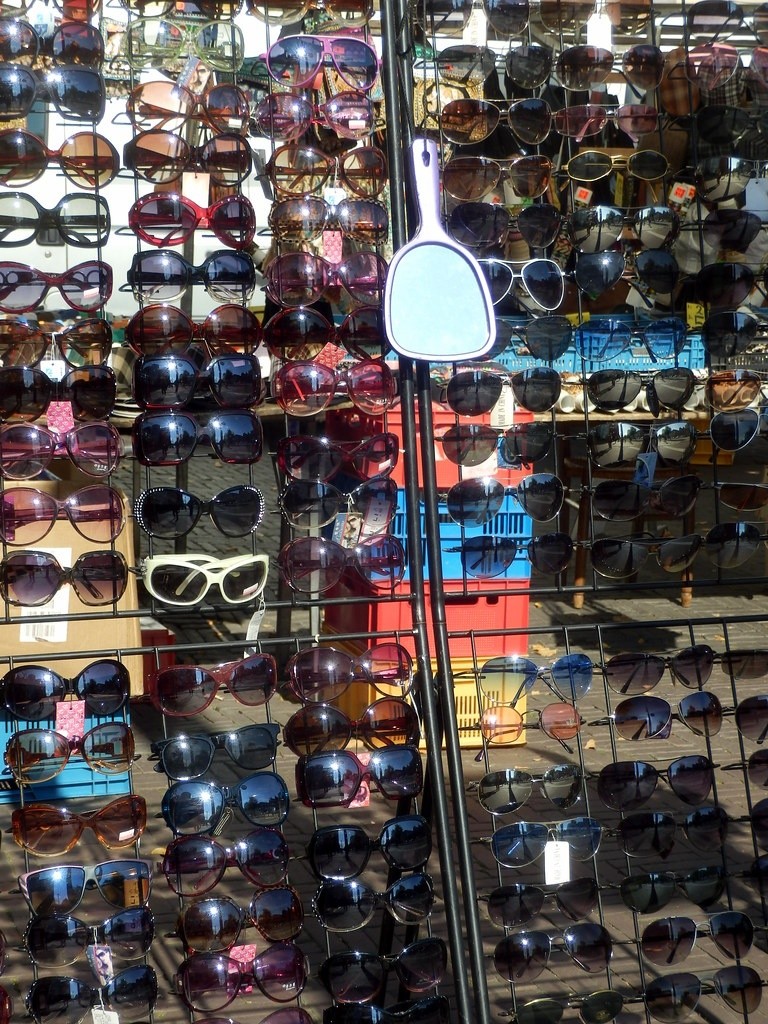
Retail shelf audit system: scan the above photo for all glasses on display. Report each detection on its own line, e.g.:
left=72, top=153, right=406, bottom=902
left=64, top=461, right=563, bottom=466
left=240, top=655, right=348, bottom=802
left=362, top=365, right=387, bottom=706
left=1, top=0, right=768, bottom=1024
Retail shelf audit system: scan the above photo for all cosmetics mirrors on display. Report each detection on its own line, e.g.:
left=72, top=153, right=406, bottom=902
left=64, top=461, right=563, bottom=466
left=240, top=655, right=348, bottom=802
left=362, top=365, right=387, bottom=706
left=380, top=138, right=497, bottom=361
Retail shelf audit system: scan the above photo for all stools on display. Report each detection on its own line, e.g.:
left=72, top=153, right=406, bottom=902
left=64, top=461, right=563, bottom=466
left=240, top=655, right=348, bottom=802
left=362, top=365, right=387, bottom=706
left=554, top=455, right=698, bottom=608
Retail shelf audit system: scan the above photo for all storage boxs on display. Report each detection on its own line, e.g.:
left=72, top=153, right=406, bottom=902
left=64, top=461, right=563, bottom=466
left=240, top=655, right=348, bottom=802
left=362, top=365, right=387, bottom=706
left=0, top=479, right=147, bottom=699
left=335, top=313, right=705, bottom=376
left=317, top=404, right=539, bottom=749
left=0, top=700, right=131, bottom=806
